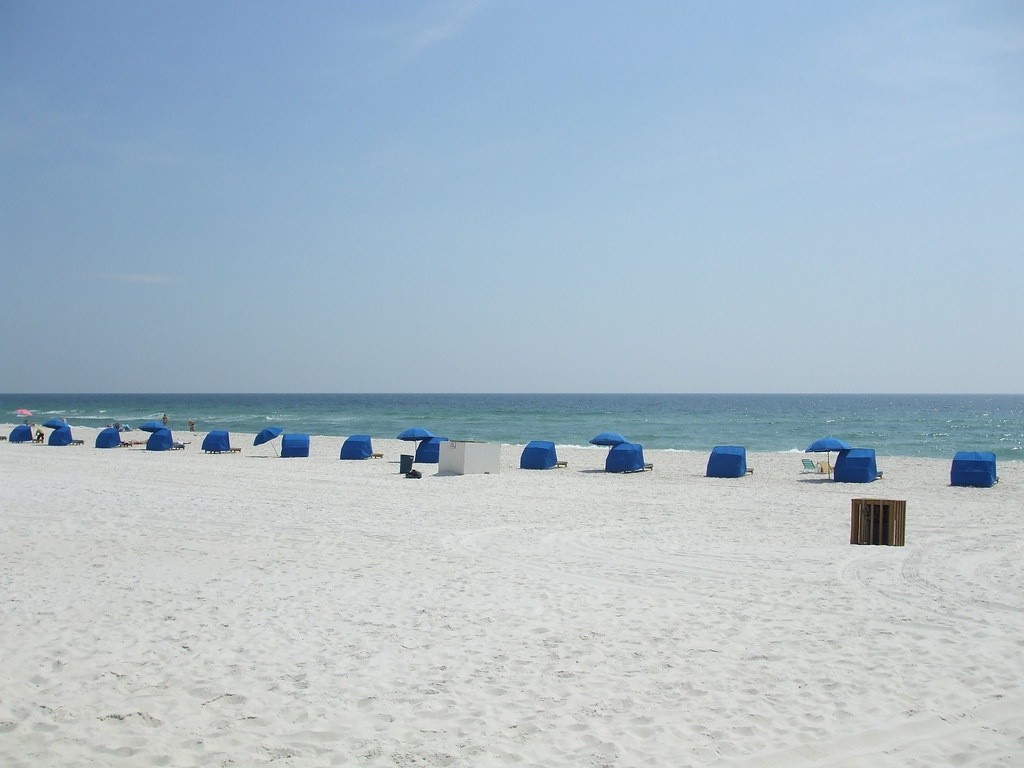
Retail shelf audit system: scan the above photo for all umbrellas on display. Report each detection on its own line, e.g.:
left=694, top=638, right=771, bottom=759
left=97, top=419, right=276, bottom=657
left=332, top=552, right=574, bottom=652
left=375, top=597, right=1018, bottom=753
left=589, top=432, right=630, bottom=451
left=16, top=409, right=33, bottom=416
left=42, top=419, right=66, bottom=429
left=805, top=437, right=853, bottom=478
left=397, top=428, right=434, bottom=457
left=138, top=421, right=167, bottom=433
left=254, top=427, right=284, bottom=455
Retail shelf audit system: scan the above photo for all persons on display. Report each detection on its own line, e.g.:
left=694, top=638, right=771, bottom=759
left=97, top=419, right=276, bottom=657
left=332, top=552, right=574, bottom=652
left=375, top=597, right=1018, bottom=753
left=36, top=427, right=45, bottom=443
left=107, top=421, right=120, bottom=429
left=61, top=417, right=66, bottom=424
left=161, top=414, right=168, bottom=426
left=188, top=418, right=195, bottom=432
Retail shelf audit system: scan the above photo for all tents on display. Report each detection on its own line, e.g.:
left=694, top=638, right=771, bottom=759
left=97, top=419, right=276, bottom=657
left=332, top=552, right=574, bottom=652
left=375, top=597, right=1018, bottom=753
left=6, top=424, right=33, bottom=442
left=144, top=429, right=174, bottom=451
left=283, top=434, right=310, bottom=457
left=950, top=452, right=997, bottom=487
left=706, top=446, right=746, bottom=478
left=48, top=429, right=74, bottom=446
left=93, top=427, right=122, bottom=448
left=606, top=444, right=644, bottom=472
left=520, top=441, right=557, bottom=468
left=415, top=436, right=448, bottom=463
left=201, top=429, right=231, bottom=452
left=833, top=447, right=877, bottom=483
left=339, top=435, right=373, bottom=460
